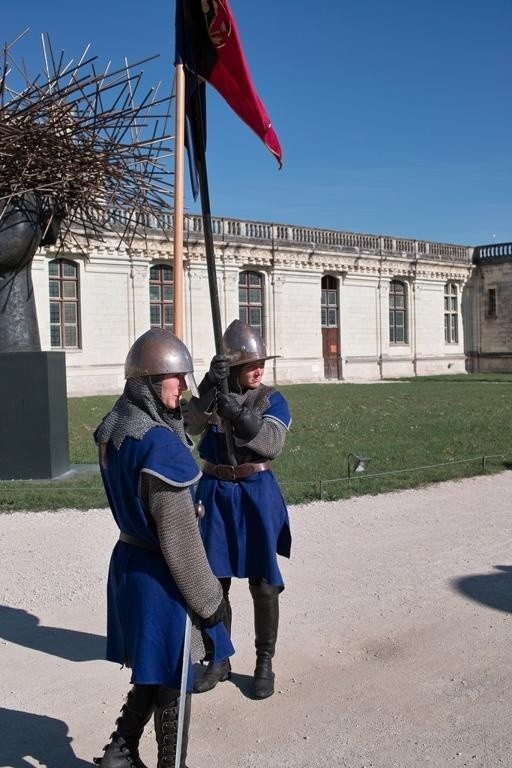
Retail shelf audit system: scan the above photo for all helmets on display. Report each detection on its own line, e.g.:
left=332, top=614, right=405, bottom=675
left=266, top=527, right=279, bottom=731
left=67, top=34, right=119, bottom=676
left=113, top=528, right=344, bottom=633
left=125, top=327, right=201, bottom=401
left=216, top=319, right=281, bottom=367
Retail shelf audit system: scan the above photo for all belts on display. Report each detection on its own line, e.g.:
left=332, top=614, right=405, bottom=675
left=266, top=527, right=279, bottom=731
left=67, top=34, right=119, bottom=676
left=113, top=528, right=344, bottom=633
left=118, top=530, right=148, bottom=549
left=201, top=458, right=272, bottom=482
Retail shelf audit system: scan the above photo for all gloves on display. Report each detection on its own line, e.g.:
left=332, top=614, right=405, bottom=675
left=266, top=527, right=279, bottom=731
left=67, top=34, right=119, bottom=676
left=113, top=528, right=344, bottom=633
left=197, top=598, right=227, bottom=629
left=209, top=355, right=229, bottom=386
left=215, top=391, right=243, bottom=422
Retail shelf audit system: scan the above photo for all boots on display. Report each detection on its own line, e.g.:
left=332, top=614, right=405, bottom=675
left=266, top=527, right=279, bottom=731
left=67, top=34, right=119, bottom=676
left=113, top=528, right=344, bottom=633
left=194, top=594, right=232, bottom=692
left=252, top=583, right=285, bottom=677
left=94, top=685, right=154, bottom=768
left=154, top=693, right=190, bottom=767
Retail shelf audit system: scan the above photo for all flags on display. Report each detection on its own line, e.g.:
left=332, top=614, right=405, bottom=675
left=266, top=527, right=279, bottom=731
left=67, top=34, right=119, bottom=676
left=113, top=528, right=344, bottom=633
left=174, top=1, right=285, bottom=172
left=186, top=69, right=206, bottom=201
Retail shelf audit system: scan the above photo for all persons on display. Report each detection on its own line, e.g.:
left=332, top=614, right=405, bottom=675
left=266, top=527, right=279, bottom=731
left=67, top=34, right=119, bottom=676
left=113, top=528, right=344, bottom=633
left=185, top=319, right=291, bottom=700
left=94, top=327, right=235, bottom=767
